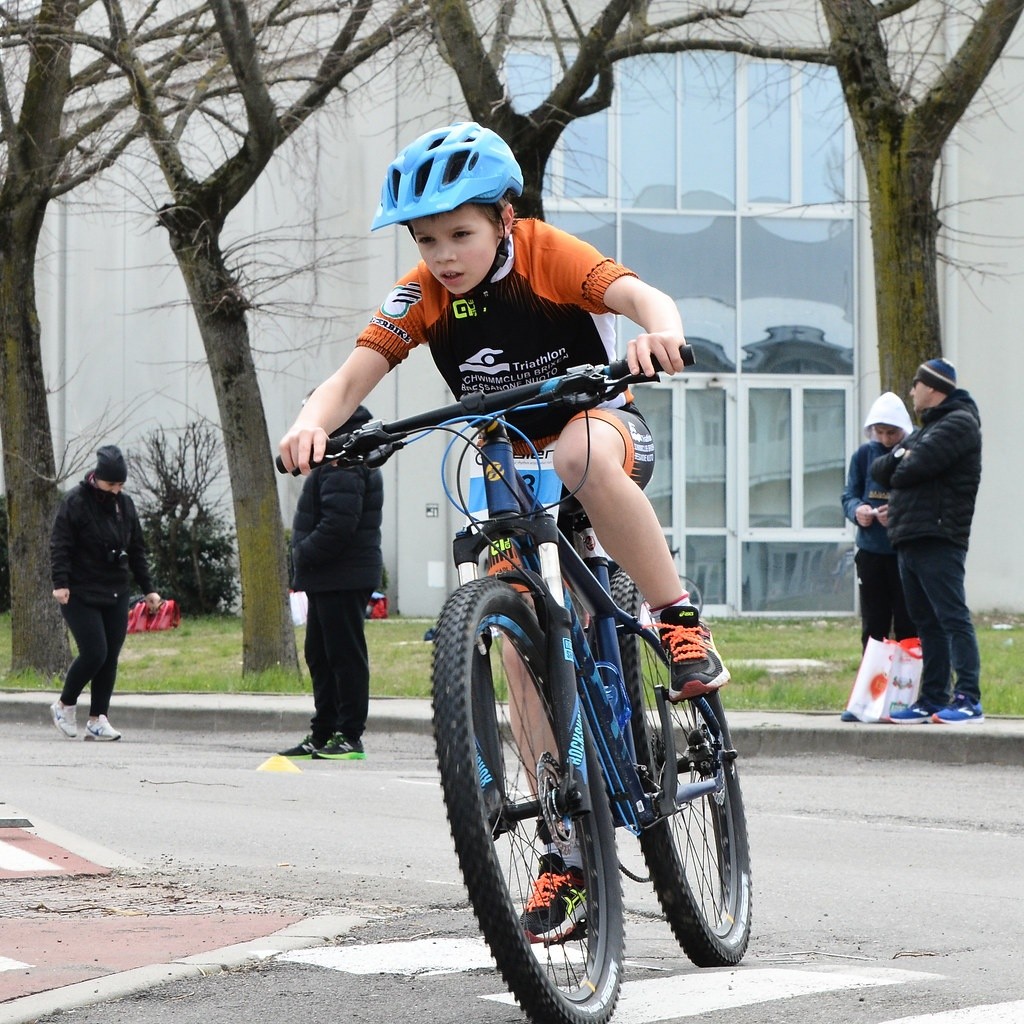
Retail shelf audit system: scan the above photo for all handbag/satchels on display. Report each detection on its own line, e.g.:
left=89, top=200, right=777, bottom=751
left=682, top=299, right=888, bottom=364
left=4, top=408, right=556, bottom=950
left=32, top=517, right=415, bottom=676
left=846, top=636, right=924, bottom=723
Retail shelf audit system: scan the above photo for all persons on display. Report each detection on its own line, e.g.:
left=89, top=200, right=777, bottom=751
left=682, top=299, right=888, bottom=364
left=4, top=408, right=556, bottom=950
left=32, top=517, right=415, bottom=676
left=50, top=446, right=161, bottom=741
left=280, top=122, right=730, bottom=939
left=281, top=390, right=384, bottom=760
left=871, top=359, right=984, bottom=722
left=841, top=394, right=918, bottom=720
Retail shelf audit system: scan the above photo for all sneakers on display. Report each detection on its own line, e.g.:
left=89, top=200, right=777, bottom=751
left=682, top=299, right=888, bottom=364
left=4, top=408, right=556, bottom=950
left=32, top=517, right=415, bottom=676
left=312, top=731, right=365, bottom=760
left=519, top=853, right=588, bottom=944
left=658, top=607, right=730, bottom=702
left=49, top=698, right=78, bottom=738
left=932, top=695, right=986, bottom=724
left=86, top=714, right=121, bottom=741
left=279, top=736, right=318, bottom=760
left=893, top=699, right=941, bottom=724
left=840, top=709, right=859, bottom=722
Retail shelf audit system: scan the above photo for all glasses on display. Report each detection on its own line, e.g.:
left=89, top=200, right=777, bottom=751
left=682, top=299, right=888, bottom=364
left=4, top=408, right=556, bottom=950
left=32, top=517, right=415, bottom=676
left=912, top=379, right=921, bottom=387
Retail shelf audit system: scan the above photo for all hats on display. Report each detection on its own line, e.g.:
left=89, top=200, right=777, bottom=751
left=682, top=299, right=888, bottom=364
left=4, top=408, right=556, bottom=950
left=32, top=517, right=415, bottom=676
left=918, top=358, right=957, bottom=393
left=95, top=445, right=128, bottom=483
left=371, top=122, right=523, bottom=231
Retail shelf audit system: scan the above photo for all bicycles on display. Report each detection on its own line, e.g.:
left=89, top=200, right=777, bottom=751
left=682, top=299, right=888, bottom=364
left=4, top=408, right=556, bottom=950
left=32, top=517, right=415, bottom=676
left=271, top=345, right=756, bottom=1024
